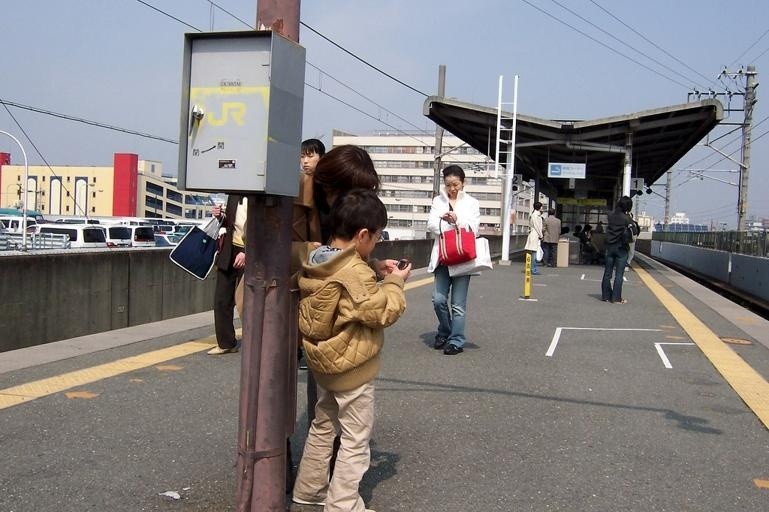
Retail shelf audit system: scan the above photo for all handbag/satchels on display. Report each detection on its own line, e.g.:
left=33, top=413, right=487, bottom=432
left=446, top=225, right=494, bottom=279
left=437, top=213, right=478, bottom=265
left=168, top=209, right=226, bottom=282
left=622, top=221, right=638, bottom=244
left=535, top=238, right=545, bottom=263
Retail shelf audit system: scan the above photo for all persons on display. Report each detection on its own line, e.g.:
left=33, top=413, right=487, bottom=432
left=206, top=191, right=249, bottom=355
left=538, top=207, right=604, bottom=268
left=425, top=164, right=481, bottom=356
left=287, top=194, right=412, bottom=511
left=287, top=144, right=384, bottom=494
left=524, top=201, right=544, bottom=275
left=289, top=137, right=326, bottom=177
left=624, top=211, right=640, bottom=271
left=601, top=195, right=639, bottom=306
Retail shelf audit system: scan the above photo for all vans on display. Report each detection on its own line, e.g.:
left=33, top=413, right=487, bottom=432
left=0, top=207, right=219, bottom=251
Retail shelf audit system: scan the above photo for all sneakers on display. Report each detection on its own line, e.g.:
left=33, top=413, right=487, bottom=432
left=603, top=296, right=628, bottom=305
left=291, top=492, right=328, bottom=506
left=443, top=344, right=463, bottom=356
left=207, top=342, right=240, bottom=355
left=433, top=333, right=448, bottom=350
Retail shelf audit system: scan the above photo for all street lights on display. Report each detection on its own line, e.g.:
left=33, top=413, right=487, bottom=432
left=388, top=216, right=393, bottom=228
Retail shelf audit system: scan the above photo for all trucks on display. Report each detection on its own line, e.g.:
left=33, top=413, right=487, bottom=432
left=382, top=229, right=418, bottom=240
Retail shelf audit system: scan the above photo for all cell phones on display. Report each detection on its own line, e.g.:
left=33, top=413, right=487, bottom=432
left=396, top=259, right=409, bottom=271
